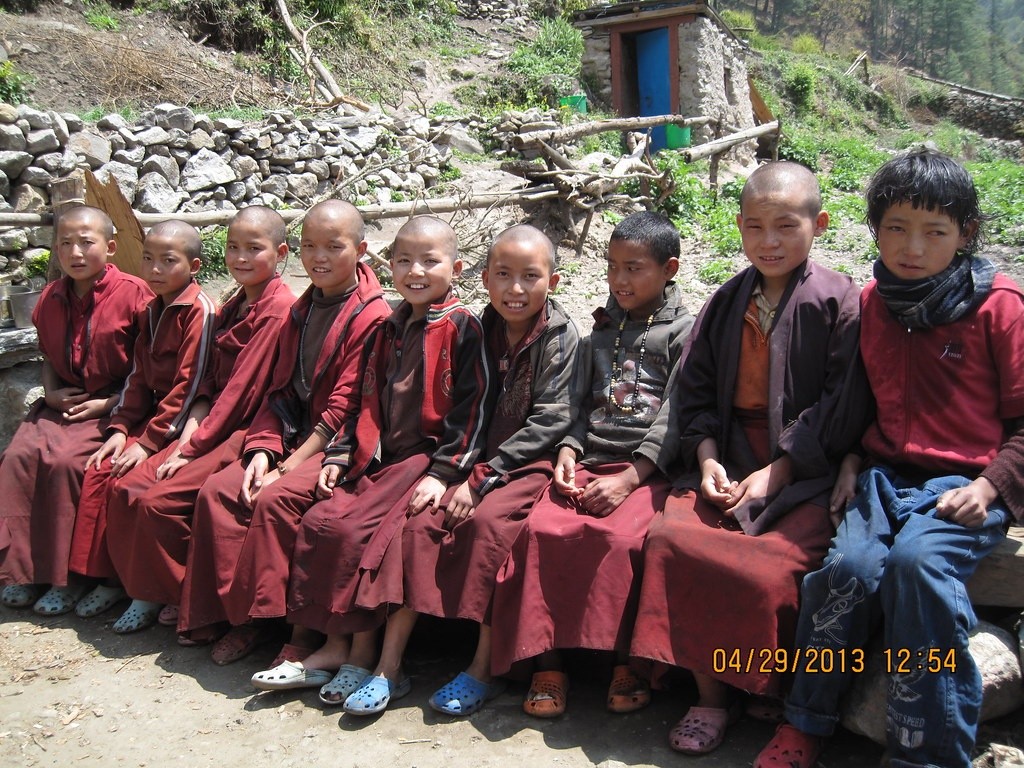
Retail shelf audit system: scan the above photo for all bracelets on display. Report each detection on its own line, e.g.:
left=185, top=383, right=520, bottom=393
left=277, top=461, right=288, bottom=475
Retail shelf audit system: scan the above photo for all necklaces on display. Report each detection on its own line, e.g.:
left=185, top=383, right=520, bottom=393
left=300, top=303, right=314, bottom=391
left=608, top=306, right=663, bottom=413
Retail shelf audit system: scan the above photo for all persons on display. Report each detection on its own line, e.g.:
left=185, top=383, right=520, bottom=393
left=74, top=200, right=487, bottom=702
left=0, top=204, right=156, bottom=614
left=753, top=146, right=1024, bottom=768
left=630, top=162, right=864, bottom=753
left=489, top=214, right=698, bottom=715
left=346, top=225, right=583, bottom=717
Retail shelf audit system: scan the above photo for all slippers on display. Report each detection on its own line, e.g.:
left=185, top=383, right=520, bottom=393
left=319, top=665, right=373, bottom=704
left=177, top=625, right=226, bottom=646
left=669, top=707, right=739, bottom=753
left=607, top=666, right=649, bottom=713
left=429, top=671, right=506, bottom=715
left=343, top=674, right=410, bottom=715
left=1, top=584, right=42, bottom=607
left=250, top=659, right=333, bottom=690
left=753, top=723, right=824, bottom=768
left=748, top=695, right=786, bottom=722
left=113, top=598, right=160, bottom=633
left=34, top=586, right=85, bottom=616
left=210, top=622, right=275, bottom=666
left=263, top=645, right=311, bottom=670
left=158, top=599, right=180, bottom=626
left=524, top=670, right=569, bottom=718
left=75, top=585, right=124, bottom=617
